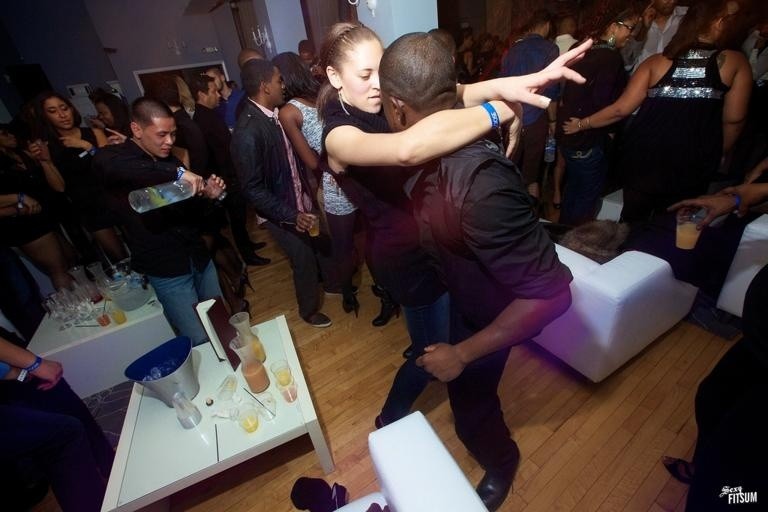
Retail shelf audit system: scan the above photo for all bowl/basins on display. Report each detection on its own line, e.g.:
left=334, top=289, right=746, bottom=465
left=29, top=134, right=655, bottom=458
left=124, top=335, right=200, bottom=407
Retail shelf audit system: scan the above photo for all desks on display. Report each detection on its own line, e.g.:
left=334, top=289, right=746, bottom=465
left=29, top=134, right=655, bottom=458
left=21, top=263, right=179, bottom=406
left=95, top=313, right=335, bottom=512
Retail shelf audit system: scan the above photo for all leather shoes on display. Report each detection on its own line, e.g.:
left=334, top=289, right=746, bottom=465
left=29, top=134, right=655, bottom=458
left=256, top=241, right=267, bottom=250
left=474, top=446, right=521, bottom=512
left=247, top=254, right=271, bottom=266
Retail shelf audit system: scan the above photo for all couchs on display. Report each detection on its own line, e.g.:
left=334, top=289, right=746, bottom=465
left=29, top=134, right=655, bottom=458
left=592, top=179, right=767, bottom=317
left=325, top=407, right=496, bottom=512
left=522, top=230, right=696, bottom=386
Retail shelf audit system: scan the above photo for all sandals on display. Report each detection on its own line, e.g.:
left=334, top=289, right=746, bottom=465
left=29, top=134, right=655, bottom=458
left=660, top=454, right=693, bottom=484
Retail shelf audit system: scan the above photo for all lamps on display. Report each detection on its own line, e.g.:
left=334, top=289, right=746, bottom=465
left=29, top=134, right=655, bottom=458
left=347, top=0, right=379, bottom=19
left=248, top=23, right=273, bottom=57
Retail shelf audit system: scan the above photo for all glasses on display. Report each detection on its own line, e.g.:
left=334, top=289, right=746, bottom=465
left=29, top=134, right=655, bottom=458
left=617, top=21, right=637, bottom=31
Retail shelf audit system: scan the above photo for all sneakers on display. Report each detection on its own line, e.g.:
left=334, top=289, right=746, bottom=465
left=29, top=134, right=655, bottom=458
left=304, top=312, right=333, bottom=329
left=322, top=284, right=360, bottom=295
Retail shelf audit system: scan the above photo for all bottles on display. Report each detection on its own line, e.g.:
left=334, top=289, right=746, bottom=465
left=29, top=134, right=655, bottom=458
left=544, top=129, right=557, bottom=162
left=215, top=373, right=236, bottom=400
left=228, top=336, right=271, bottom=394
left=128, top=178, right=197, bottom=215
left=228, top=311, right=267, bottom=364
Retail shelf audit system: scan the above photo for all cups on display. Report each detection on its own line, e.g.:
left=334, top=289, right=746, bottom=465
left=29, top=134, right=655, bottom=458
left=675, top=214, right=705, bottom=250
left=252, top=393, right=276, bottom=422
left=306, top=215, right=319, bottom=237
left=170, top=392, right=202, bottom=431
left=33, top=139, right=49, bottom=161
left=42, top=262, right=151, bottom=329
left=270, top=359, right=297, bottom=403
left=202, top=176, right=228, bottom=201
left=232, top=403, right=259, bottom=433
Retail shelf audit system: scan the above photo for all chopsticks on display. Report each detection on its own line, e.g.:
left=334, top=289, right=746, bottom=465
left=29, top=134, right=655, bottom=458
left=213, top=424, right=219, bottom=464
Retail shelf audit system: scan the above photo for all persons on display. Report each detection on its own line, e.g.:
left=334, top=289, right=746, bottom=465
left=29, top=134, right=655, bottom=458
left=2, top=0, right=768, bottom=511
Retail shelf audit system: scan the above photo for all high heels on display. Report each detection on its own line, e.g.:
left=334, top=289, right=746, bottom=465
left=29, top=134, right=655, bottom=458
left=230, top=299, right=252, bottom=320
left=341, top=293, right=361, bottom=319
left=234, top=274, right=254, bottom=299
left=372, top=300, right=402, bottom=327
